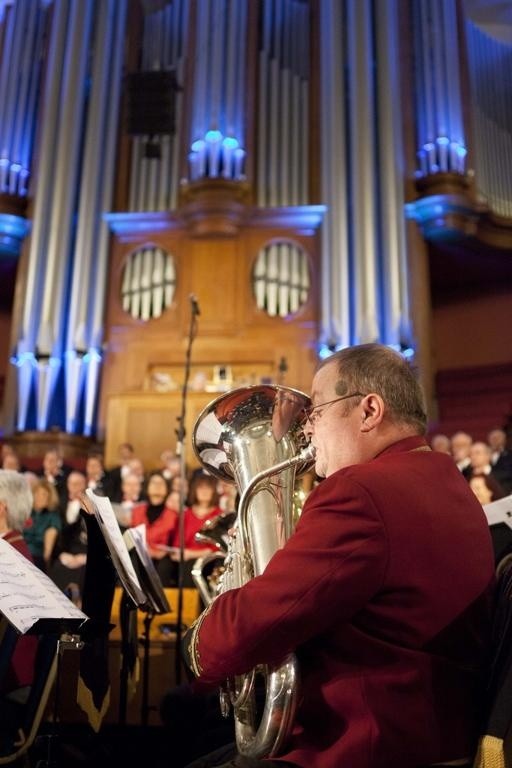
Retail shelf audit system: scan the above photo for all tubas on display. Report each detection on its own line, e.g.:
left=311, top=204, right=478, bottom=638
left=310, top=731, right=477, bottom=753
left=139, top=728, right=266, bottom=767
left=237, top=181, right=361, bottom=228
left=190, top=382, right=315, bottom=759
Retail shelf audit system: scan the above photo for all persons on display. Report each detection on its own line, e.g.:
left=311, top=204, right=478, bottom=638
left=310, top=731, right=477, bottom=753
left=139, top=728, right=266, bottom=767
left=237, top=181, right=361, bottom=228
left=2, top=444, right=241, bottom=687
left=181, top=345, right=494, bottom=768
left=430, top=428, right=512, bottom=507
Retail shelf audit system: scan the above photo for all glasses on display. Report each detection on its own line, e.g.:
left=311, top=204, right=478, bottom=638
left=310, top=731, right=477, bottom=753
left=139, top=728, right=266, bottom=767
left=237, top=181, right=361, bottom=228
left=303, top=392, right=366, bottom=425
